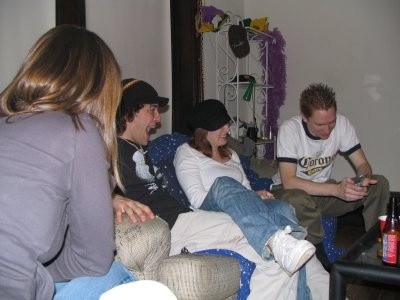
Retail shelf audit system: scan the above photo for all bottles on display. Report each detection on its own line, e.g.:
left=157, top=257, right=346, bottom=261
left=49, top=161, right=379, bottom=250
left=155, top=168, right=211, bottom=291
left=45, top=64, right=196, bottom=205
left=382, top=196, right=400, bottom=267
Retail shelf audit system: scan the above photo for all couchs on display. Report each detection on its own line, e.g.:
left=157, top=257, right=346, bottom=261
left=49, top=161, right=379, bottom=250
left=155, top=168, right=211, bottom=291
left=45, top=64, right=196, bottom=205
left=115, top=132, right=346, bottom=300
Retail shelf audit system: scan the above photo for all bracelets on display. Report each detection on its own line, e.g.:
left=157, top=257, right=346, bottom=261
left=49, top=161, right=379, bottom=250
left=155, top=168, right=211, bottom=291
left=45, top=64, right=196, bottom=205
left=358, top=174, right=368, bottom=178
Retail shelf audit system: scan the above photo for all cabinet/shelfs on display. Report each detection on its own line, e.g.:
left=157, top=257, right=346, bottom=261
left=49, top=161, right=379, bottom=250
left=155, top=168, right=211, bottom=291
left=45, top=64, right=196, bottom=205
left=215, top=10, right=276, bottom=160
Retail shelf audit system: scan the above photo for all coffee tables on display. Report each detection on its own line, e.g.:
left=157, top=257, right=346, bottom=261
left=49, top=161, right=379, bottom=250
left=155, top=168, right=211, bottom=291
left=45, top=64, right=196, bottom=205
left=329, top=220, right=400, bottom=300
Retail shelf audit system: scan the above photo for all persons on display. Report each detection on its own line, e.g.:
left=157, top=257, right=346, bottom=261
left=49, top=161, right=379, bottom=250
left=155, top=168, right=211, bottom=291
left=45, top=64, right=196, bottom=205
left=0, top=23, right=137, bottom=300
left=112, top=78, right=311, bottom=300
left=270, top=82, right=391, bottom=271
left=172, top=98, right=316, bottom=278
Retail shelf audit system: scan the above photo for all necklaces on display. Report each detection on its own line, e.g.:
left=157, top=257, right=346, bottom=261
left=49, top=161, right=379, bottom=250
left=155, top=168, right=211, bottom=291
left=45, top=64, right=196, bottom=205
left=121, top=136, right=147, bottom=156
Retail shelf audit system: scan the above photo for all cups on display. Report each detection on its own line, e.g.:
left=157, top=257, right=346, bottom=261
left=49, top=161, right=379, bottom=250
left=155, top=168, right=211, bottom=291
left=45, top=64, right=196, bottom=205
left=377, top=215, right=387, bottom=233
